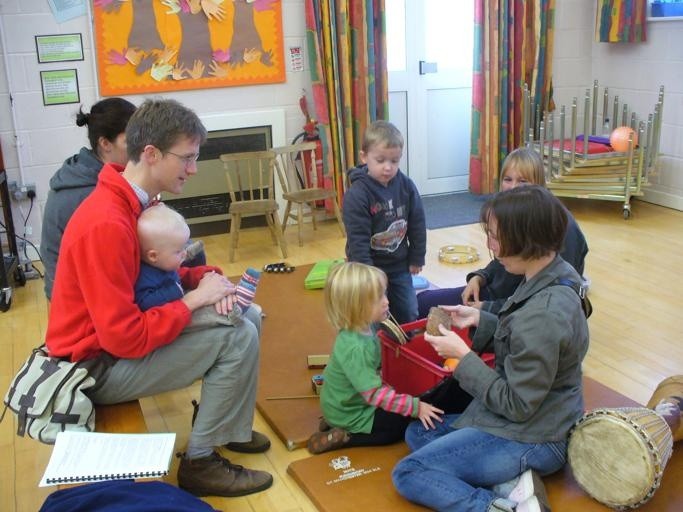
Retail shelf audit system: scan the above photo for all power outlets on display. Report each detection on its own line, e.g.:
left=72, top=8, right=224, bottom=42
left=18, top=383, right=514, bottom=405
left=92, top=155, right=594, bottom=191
left=11, top=183, right=36, bottom=203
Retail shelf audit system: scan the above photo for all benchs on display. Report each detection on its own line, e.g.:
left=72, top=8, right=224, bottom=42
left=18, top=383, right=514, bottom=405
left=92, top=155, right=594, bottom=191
left=50, top=398, right=167, bottom=511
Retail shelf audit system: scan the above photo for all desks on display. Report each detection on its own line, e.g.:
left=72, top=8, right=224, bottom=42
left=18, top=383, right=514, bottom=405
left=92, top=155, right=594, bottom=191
left=519, top=78, right=666, bottom=199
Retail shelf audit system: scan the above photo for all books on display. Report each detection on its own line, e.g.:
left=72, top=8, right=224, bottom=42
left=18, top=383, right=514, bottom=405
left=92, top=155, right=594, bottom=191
left=36, top=428, right=176, bottom=489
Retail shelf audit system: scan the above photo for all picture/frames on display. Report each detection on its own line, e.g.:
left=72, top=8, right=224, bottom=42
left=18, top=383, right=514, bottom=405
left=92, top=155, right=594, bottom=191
left=644, top=1, right=683, bottom=21
left=88, top=1, right=286, bottom=99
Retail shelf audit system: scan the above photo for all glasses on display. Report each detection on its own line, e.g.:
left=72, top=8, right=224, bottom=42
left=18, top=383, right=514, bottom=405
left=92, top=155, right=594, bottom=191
left=165, top=153, right=200, bottom=163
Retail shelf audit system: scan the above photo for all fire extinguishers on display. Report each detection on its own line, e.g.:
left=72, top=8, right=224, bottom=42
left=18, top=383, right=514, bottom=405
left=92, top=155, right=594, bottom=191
left=289, top=118, right=325, bottom=208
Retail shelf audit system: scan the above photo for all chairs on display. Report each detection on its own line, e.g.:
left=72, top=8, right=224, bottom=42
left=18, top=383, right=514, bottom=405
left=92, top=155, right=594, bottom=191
left=219, top=150, right=288, bottom=260
left=266, top=141, right=347, bottom=248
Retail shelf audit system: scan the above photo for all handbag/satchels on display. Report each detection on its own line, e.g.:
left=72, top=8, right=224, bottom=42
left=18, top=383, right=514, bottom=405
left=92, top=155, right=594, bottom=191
left=1, top=343, right=105, bottom=444
left=414, top=373, right=472, bottom=412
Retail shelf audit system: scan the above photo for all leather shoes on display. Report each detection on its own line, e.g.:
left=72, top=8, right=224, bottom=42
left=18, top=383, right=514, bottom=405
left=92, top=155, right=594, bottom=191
left=191, top=399, right=270, bottom=453
left=176, top=450, right=273, bottom=497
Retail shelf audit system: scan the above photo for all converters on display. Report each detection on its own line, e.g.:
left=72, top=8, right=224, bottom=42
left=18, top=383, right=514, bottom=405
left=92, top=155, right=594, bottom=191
left=24, top=259, right=32, bottom=272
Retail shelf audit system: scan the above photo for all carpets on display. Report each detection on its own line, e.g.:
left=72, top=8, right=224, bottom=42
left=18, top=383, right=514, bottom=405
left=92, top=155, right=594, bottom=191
left=419, top=189, right=494, bottom=230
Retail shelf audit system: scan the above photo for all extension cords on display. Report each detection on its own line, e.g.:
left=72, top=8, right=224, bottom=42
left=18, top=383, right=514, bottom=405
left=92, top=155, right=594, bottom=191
left=15, top=271, right=39, bottom=279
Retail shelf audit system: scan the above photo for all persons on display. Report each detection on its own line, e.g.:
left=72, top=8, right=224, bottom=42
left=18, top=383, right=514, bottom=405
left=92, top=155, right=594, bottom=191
left=37, top=95, right=206, bottom=299
left=389, top=184, right=592, bottom=512
left=303, top=261, right=446, bottom=454
left=45, top=99, right=275, bottom=498
left=132, top=204, right=260, bottom=335
left=416, top=147, right=589, bottom=318
left=339, top=120, right=429, bottom=340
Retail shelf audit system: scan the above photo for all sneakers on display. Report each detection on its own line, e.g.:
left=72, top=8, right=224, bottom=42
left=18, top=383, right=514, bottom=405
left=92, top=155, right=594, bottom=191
left=318, top=416, right=330, bottom=432
left=308, top=427, right=351, bottom=454
left=491, top=468, right=550, bottom=511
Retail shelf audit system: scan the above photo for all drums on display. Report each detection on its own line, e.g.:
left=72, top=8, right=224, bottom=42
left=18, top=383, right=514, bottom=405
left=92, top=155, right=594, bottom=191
left=566, top=374, right=683, bottom=511
